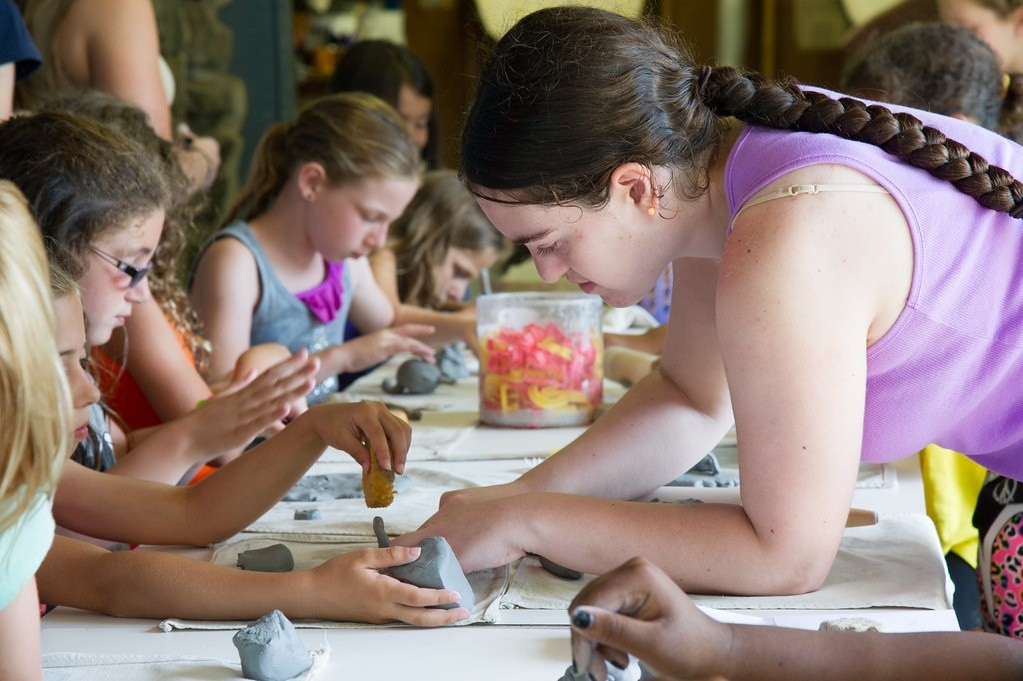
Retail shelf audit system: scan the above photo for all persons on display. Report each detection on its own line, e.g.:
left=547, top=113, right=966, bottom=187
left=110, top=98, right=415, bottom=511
left=836, top=20, right=1022, bottom=631
left=599, top=261, right=672, bottom=388
left=20, top=0, right=223, bottom=208
left=316, top=38, right=436, bottom=170
left=0, top=1, right=43, bottom=123
left=17, top=90, right=309, bottom=472
left=188, top=91, right=436, bottom=417
left=378, top=8, right=1023, bottom=639
left=0, top=108, right=321, bottom=552
left=567, top=557, right=1023, bottom=681
left=0, top=180, right=74, bottom=681
left=364, top=169, right=515, bottom=360
left=31, top=234, right=472, bottom=628
left=928, top=0, right=1023, bottom=144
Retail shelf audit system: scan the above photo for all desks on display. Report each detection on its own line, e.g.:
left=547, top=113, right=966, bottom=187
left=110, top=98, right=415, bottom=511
left=39, top=328, right=962, bottom=681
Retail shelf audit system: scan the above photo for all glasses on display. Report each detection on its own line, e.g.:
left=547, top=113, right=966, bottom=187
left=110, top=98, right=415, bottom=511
left=89, top=244, right=154, bottom=287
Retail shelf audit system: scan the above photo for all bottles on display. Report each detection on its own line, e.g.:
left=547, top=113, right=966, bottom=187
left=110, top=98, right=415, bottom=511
left=477, top=293, right=603, bottom=428
